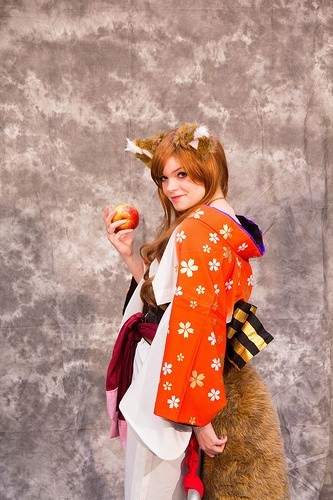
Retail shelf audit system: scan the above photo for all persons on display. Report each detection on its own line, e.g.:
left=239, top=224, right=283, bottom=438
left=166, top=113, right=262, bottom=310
left=101, top=124, right=291, bottom=499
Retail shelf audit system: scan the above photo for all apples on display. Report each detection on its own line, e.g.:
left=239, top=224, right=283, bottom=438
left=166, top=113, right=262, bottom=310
left=112, top=205, right=139, bottom=231
